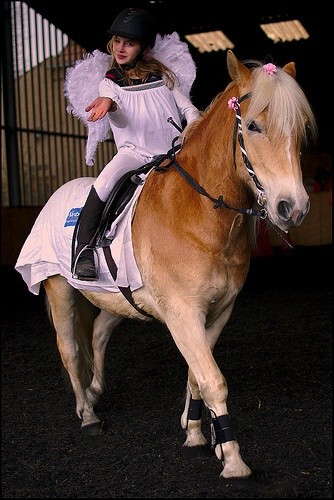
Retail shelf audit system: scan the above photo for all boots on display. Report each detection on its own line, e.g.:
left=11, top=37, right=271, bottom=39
left=74, top=185, right=107, bottom=280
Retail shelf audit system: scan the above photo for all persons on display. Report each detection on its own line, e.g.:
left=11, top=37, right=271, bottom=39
left=73, top=10, right=202, bottom=281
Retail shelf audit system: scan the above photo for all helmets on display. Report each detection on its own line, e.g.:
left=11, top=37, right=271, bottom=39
left=108, top=7, right=156, bottom=49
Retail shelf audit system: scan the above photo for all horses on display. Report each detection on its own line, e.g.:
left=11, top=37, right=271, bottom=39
left=38, top=49, right=319, bottom=480
